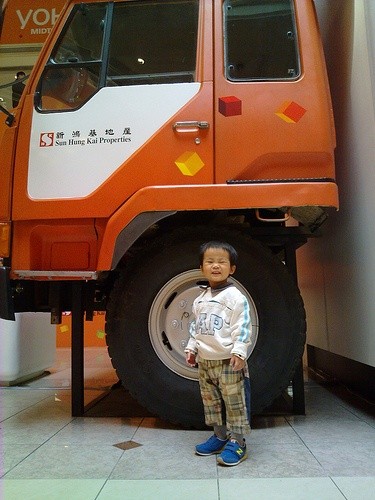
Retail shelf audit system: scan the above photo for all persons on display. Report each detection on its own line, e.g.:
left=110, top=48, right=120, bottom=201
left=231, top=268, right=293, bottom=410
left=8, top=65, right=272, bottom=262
left=12, top=72, right=27, bottom=108
left=185, top=240, right=252, bottom=465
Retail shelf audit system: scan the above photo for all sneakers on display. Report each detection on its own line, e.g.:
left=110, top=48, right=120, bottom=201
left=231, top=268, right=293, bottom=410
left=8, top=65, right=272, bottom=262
left=216, top=439, right=248, bottom=466
left=194, top=435, right=229, bottom=456
